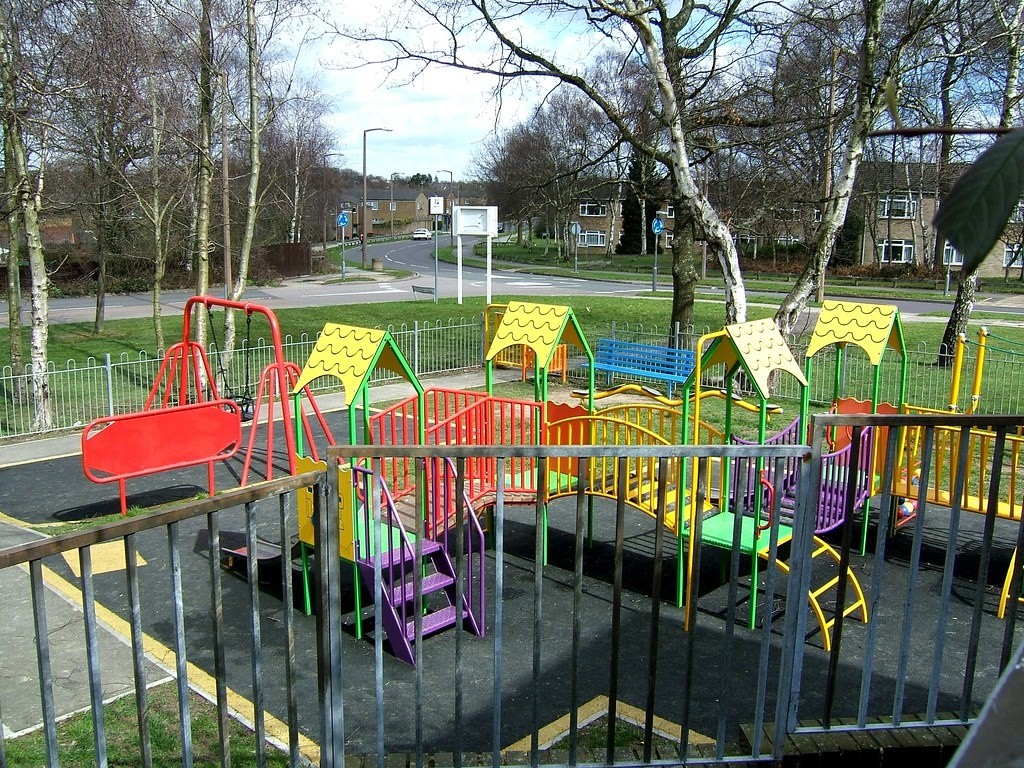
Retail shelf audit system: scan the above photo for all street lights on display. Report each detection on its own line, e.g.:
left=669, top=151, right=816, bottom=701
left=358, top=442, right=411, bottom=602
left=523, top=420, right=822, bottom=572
left=321, top=152, right=345, bottom=253
left=945, top=243, right=954, bottom=291
left=437, top=169, right=454, bottom=244
left=361, top=127, right=393, bottom=266
left=571, top=221, right=578, bottom=272
left=816, top=46, right=856, bottom=301
left=390, top=172, right=404, bottom=240
left=653, top=209, right=667, bottom=292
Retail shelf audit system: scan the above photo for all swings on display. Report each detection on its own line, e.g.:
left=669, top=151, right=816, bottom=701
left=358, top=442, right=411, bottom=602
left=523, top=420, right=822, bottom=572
left=203, top=295, right=256, bottom=422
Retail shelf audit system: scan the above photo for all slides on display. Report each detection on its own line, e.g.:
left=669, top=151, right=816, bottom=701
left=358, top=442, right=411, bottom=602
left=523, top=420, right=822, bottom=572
left=221, top=538, right=315, bottom=567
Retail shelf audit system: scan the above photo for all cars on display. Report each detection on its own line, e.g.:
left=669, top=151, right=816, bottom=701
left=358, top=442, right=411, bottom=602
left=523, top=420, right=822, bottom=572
left=412, top=228, right=432, bottom=240
left=499, top=222, right=504, bottom=233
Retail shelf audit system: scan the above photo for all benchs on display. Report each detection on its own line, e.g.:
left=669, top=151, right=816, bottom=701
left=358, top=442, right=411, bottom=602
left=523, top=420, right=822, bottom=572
left=581, top=338, right=703, bottom=399
left=412, top=284, right=436, bottom=303
